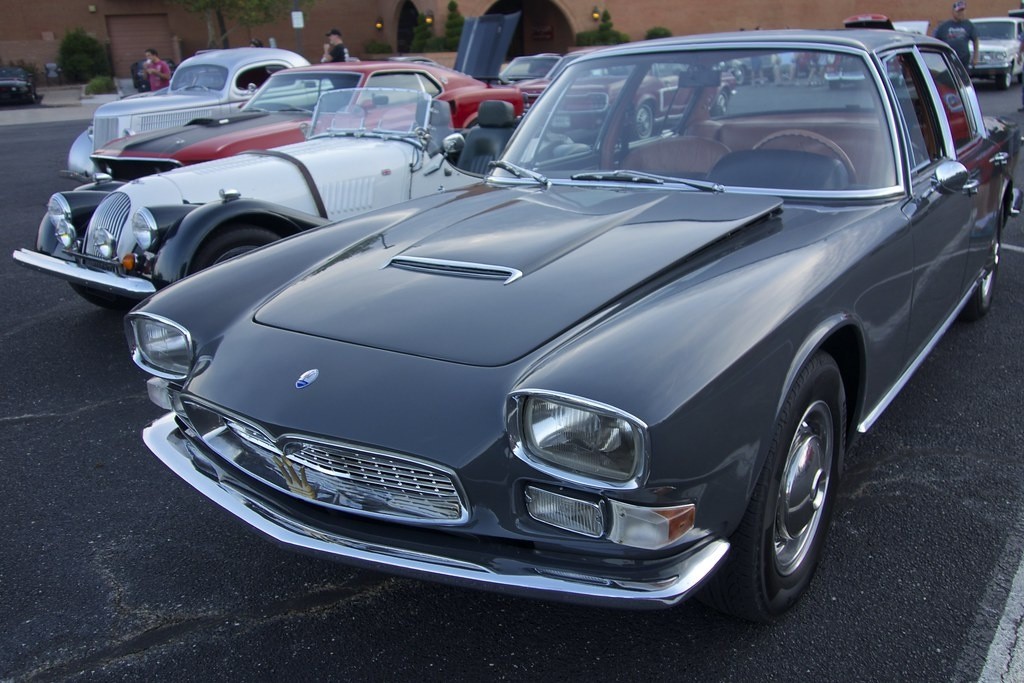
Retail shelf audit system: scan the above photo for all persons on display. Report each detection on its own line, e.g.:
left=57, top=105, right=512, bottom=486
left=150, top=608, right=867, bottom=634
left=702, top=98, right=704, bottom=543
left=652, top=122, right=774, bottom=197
left=740, top=26, right=842, bottom=87
left=143, top=49, right=170, bottom=91
left=323, top=28, right=349, bottom=88
left=250, top=38, right=263, bottom=47
left=932, top=2, right=979, bottom=75
left=1016, top=31, right=1024, bottom=113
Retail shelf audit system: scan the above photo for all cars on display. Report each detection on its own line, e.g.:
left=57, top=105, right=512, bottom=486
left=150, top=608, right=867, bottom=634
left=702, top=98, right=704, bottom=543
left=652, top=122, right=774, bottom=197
left=967, top=17, right=1024, bottom=90
left=87, top=60, right=531, bottom=184
left=13, top=85, right=630, bottom=313
left=520, top=46, right=738, bottom=144
left=824, top=13, right=916, bottom=88
left=498, top=47, right=611, bottom=85
left=0, top=67, right=37, bottom=105
left=612, top=59, right=750, bottom=87
left=58, top=47, right=335, bottom=183
left=127, top=27, right=1022, bottom=621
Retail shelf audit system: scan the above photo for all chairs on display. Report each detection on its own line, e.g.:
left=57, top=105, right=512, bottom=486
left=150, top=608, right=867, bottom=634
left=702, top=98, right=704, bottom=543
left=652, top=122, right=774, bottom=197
left=618, top=135, right=731, bottom=175
left=454, top=99, right=518, bottom=176
left=413, top=97, right=454, bottom=151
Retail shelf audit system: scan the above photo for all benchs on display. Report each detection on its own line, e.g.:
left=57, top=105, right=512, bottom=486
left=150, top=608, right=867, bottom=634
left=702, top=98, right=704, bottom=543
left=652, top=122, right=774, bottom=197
left=683, top=113, right=884, bottom=181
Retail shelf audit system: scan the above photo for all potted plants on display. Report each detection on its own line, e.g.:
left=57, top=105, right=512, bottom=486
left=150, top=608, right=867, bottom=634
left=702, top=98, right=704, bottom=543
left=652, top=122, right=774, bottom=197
left=80, top=75, right=125, bottom=104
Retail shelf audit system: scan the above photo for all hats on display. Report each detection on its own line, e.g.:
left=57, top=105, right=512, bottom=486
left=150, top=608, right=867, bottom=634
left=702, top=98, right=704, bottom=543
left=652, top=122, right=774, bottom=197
left=326, top=28, right=342, bottom=36
left=952, top=1, right=966, bottom=11
